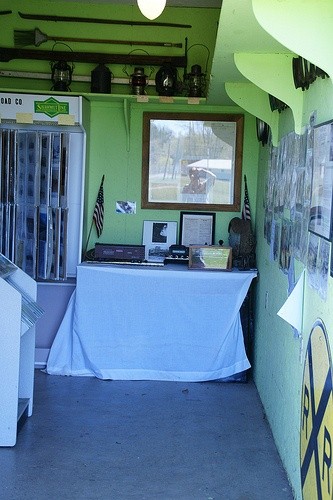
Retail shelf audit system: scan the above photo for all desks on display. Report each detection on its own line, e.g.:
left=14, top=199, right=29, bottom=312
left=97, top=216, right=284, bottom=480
left=46, top=261, right=258, bottom=380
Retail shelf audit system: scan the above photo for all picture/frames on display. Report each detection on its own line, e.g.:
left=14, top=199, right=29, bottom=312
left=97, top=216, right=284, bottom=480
left=189, top=244, right=233, bottom=272
left=141, top=112, right=245, bottom=212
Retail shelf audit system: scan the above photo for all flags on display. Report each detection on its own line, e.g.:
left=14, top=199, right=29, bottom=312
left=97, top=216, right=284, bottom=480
left=242, top=180, right=251, bottom=222
left=93, top=182, right=105, bottom=238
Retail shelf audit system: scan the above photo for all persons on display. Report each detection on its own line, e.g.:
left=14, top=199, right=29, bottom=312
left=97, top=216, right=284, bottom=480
left=182, top=168, right=216, bottom=203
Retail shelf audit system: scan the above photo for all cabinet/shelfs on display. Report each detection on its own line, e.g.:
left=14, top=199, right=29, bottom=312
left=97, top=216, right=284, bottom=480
left=0, top=252, right=36, bottom=447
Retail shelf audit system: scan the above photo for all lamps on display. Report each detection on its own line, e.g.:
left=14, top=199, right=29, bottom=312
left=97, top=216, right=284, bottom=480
left=48, top=42, right=74, bottom=92
left=181, top=44, right=211, bottom=97
left=122, top=48, right=155, bottom=95
